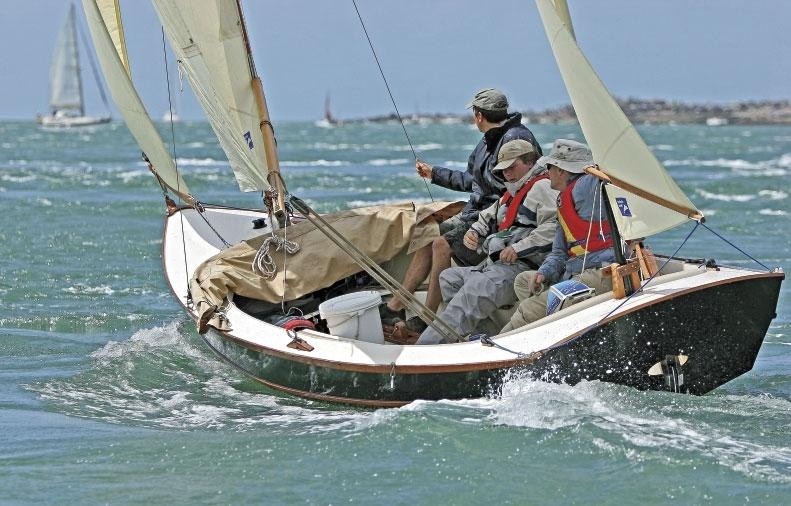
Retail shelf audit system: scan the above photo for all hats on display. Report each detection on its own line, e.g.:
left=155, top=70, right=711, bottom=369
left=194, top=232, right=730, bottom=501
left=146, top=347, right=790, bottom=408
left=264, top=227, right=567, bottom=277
left=536, top=139, right=594, bottom=174
left=490, top=139, right=535, bottom=174
left=465, top=88, right=509, bottom=111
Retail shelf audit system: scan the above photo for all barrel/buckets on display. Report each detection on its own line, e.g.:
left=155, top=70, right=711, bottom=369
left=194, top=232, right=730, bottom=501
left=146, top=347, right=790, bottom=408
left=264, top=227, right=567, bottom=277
left=318, top=290, right=386, bottom=346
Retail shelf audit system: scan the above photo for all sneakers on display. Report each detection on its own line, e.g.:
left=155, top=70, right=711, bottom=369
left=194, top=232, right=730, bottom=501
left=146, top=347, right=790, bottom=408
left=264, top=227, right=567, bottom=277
left=379, top=303, right=406, bottom=326
left=407, top=316, right=428, bottom=334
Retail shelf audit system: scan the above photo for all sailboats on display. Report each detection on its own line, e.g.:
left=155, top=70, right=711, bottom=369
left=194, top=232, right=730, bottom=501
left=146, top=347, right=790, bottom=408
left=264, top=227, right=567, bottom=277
left=76, top=0, right=788, bottom=421
left=314, top=92, right=338, bottom=129
left=34, top=0, right=113, bottom=127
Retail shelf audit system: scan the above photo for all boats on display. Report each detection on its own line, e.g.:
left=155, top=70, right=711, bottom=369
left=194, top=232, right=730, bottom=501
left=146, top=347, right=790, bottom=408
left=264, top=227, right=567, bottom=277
left=160, top=108, right=181, bottom=124
left=706, top=114, right=728, bottom=127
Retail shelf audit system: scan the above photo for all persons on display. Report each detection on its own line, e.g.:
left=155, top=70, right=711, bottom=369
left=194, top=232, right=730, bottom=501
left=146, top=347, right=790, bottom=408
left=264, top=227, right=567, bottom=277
left=378, top=86, right=544, bottom=339
left=417, top=140, right=562, bottom=348
left=495, top=139, right=645, bottom=335
